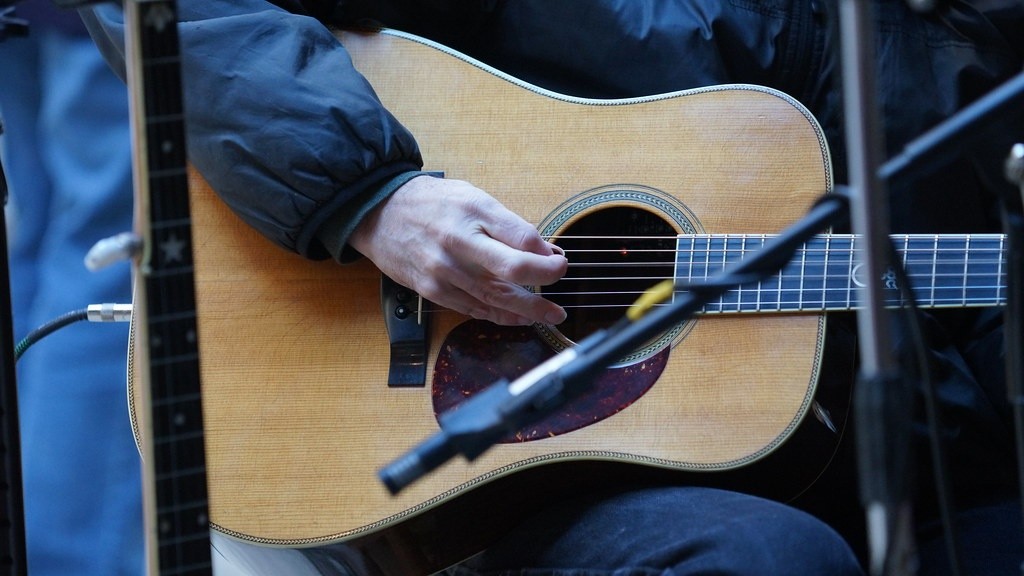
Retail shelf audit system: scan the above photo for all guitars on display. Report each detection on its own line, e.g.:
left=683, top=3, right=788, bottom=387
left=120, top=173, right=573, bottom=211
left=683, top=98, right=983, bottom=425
left=120, top=25, right=1010, bottom=576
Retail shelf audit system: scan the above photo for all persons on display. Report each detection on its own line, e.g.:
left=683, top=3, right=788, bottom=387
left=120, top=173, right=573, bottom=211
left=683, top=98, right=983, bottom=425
left=77, top=0, right=864, bottom=576
